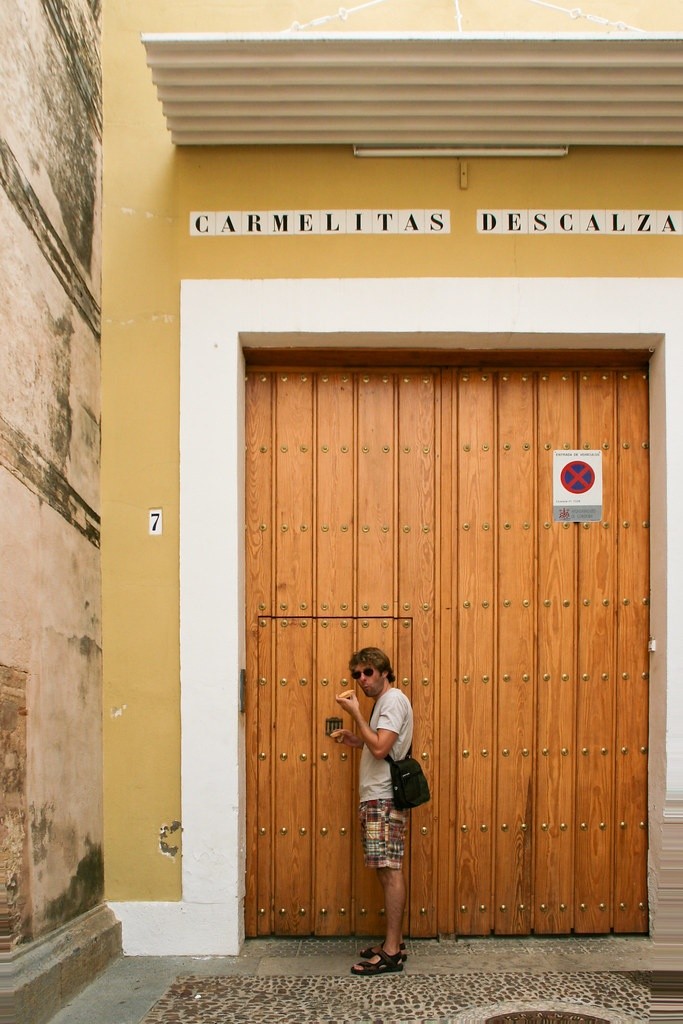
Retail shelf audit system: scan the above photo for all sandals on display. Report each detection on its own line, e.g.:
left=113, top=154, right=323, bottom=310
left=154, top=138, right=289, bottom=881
left=350, top=950, right=404, bottom=975
left=359, top=939, right=407, bottom=963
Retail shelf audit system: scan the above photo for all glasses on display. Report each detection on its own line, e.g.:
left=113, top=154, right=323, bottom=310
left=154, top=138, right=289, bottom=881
left=352, top=667, right=381, bottom=679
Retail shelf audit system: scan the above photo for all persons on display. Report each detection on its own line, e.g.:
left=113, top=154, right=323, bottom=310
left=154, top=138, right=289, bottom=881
left=330, top=646, right=414, bottom=976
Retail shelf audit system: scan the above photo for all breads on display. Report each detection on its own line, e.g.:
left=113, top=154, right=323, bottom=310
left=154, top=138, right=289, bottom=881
left=339, top=690, right=354, bottom=699
left=330, top=730, right=342, bottom=737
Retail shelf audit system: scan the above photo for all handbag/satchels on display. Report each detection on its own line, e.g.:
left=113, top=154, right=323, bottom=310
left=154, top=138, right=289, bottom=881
left=390, top=758, right=430, bottom=810
